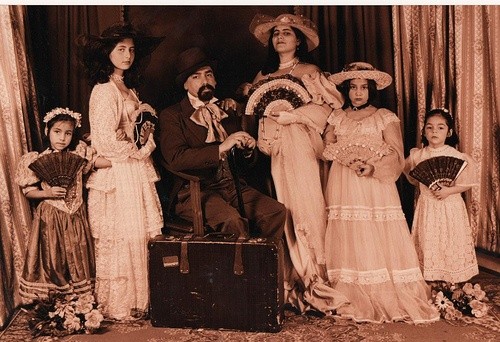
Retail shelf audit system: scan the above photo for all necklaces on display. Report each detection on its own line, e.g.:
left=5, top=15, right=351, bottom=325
left=350, top=102, right=370, bottom=110
left=278, top=57, right=299, bottom=69
left=110, top=72, right=124, bottom=83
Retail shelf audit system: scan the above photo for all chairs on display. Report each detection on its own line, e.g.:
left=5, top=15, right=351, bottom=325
left=159, top=158, right=205, bottom=235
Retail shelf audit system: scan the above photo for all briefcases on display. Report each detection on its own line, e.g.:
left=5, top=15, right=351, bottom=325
left=147, top=232, right=285, bottom=333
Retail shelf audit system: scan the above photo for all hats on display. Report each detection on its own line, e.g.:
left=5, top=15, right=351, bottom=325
left=174, top=47, right=210, bottom=80
left=81, top=24, right=166, bottom=69
left=328, top=62, right=392, bottom=91
left=249, top=12, right=320, bottom=53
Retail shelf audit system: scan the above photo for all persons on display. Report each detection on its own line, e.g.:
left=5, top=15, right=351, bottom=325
left=219, top=13, right=348, bottom=317
left=323, top=62, right=441, bottom=325
left=403, top=109, right=479, bottom=291
left=83, top=24, right=165, bottom=323
left=156, top=46, right=288, bottom=242
left=14, top=108, right=111, bottom=337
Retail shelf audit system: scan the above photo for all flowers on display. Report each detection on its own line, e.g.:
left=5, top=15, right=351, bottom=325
left=429, top=279, right=494, bottom=327
left=26, top=288, right=104, bottom=337
left=43, top=107, right=82, bottom=136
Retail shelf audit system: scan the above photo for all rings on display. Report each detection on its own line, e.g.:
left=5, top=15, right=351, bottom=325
left=243, top=136, right=246, bottom=139
